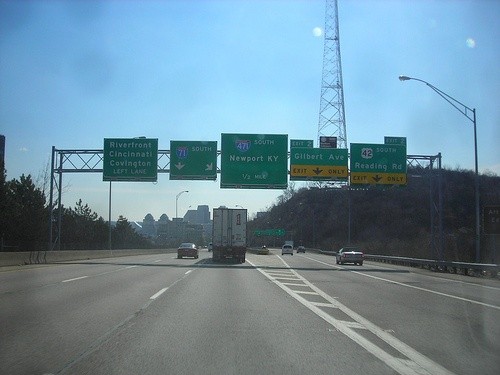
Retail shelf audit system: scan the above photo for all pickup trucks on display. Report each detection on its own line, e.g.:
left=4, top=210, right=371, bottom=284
left=336, top=246, right=364, bottom=266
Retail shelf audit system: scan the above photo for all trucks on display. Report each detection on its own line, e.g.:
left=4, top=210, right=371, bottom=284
left=212, top=206, right=248, bottom=264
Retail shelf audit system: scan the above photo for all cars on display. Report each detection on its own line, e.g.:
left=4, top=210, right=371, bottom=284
left=282, top=244, right=294, bottom=255
left=208, top=242, right=213, bottom=252
left=297, top=246, right=306, bottom=254
left=178, top=242, right=199, bottom=259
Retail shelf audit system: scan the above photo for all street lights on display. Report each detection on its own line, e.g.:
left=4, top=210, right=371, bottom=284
left=400, top=73, right=481, bottom=264
left=107, top=135, right=146, bottom=250
left=175, top=190, right=189, bottom=219
